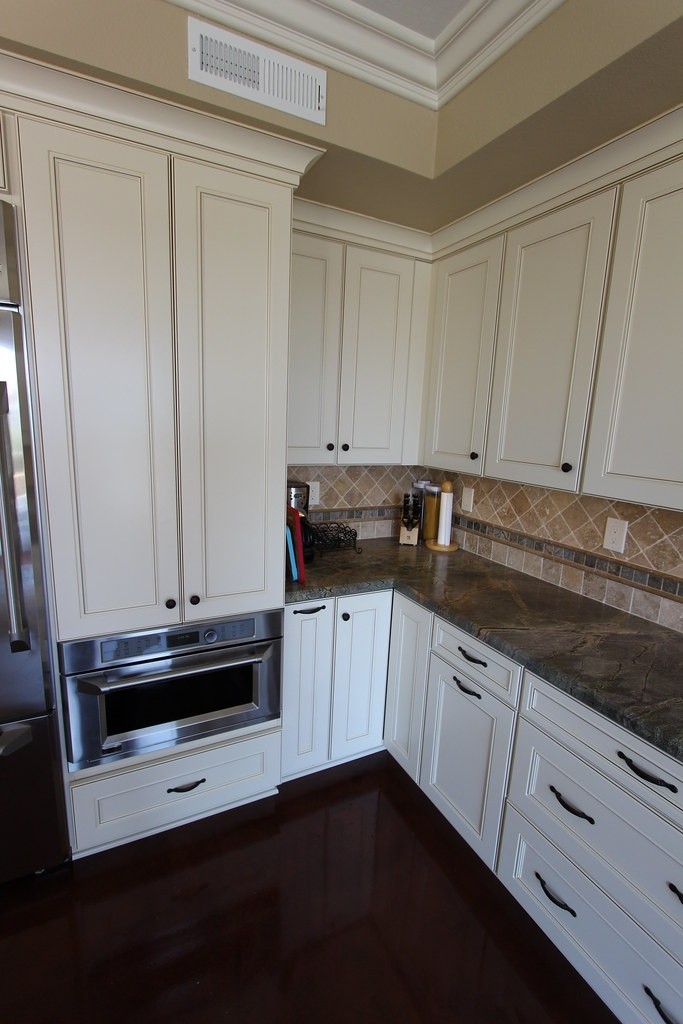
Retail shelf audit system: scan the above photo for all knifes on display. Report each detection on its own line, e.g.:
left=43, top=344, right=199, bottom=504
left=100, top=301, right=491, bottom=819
left=403, top=493, right=419, bottom=520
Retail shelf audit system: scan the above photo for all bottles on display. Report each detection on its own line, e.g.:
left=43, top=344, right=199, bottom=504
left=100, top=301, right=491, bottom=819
left=300, top=517, right=316, bottom=564
left=413, top=481, right=441, bottom=539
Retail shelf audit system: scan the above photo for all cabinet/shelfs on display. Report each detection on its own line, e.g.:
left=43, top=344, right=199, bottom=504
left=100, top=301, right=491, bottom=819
left=0, top=45, right=291, bottom=644
left=291, top=105, right=682, bottom=515
left=57, top=594, right=682, bottom=1024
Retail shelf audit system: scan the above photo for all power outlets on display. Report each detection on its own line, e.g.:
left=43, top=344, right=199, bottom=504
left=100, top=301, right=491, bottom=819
left=603, top=516, right=628, bottom=552
left=305, top=482, right=320, bottom=505
left=462, top=487, right=474, bottom=512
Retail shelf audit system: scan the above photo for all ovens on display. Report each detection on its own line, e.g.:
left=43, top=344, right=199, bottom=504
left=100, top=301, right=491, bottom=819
left=58, top=607, right=285, bottom=773
